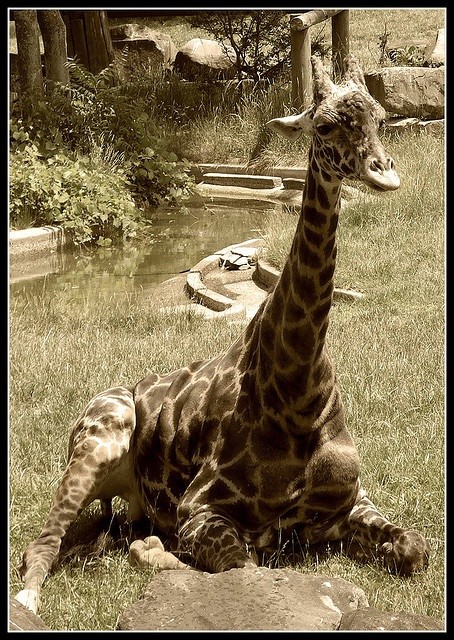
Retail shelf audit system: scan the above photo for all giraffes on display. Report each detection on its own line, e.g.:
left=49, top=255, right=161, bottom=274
left=14, top=54, right=430, bottom=615
